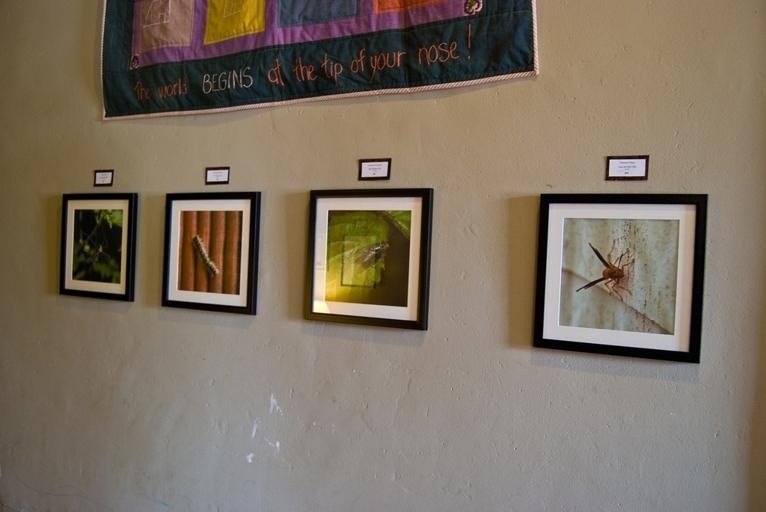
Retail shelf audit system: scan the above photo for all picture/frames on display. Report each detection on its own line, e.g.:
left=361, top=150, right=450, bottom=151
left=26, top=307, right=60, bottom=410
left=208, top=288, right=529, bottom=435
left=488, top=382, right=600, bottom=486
left=61, top=192, right=136, bottom=301
left=533, top=190, right=708, bottom=363
left=303, top=187, right=433, bottom=330
left=162, top=192, right=261, bottom=315
